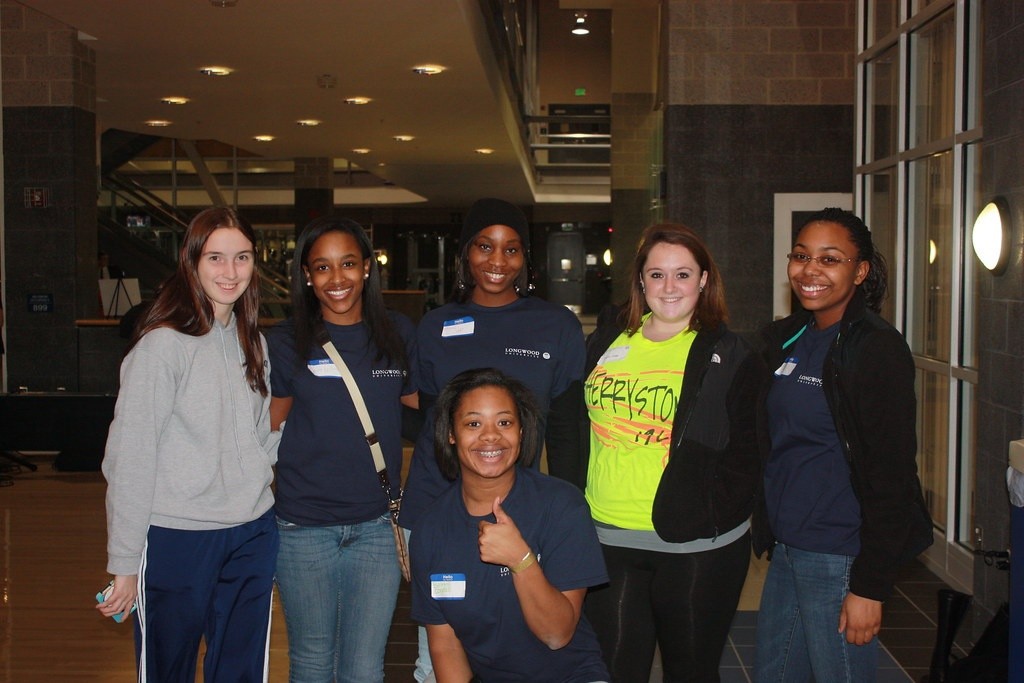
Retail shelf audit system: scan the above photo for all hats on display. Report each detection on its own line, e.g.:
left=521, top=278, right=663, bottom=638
left=457, top=196, right=537, bottom=276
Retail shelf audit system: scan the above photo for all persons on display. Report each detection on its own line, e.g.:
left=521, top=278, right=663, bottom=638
left=749, top=207, right=934, bottom=683
left=580, top=221, right=754, bottom=682
left=415, top=197, right=586, bottom=484
left=408, top=365, right=612, bottom=683
left=93, top=206, right=283, bottom=683
left=268, top=218, right=419, bottom=683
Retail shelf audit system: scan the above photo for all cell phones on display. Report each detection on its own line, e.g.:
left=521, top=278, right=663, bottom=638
left=94, top=580, right=138, bottom=624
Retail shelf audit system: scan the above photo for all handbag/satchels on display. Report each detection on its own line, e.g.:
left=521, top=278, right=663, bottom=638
left=387, top=497, right=412, bottom=583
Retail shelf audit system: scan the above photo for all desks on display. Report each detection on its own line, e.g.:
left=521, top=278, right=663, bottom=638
left=0, top=391, right=118, bottom=472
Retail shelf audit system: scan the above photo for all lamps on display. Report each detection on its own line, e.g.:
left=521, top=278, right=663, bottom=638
left=973, top=196, right=1013, bottom=276
left=571, top=10, right=590, bottom=35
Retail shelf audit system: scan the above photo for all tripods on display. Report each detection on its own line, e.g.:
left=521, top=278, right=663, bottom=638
left=107, top=278, right=135, bottom=319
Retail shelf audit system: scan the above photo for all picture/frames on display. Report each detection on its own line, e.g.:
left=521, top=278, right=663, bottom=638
left=773, top=192, right=853, bottom=322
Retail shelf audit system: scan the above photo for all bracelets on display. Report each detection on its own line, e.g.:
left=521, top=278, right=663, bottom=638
left=508, top=552, right=536, bottom=573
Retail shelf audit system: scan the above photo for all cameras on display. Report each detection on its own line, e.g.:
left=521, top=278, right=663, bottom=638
left=116, top=271, right=126, bottom=277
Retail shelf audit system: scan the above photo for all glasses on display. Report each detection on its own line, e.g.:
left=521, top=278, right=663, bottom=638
left=786, top=251, right=862, bottom=268
